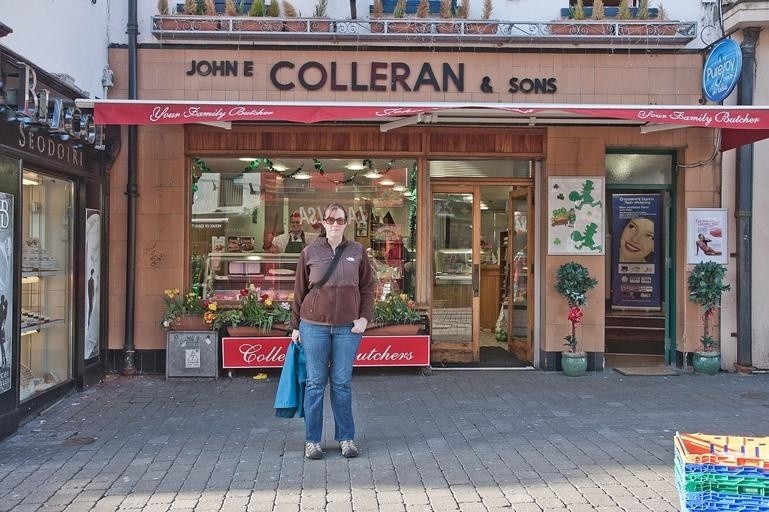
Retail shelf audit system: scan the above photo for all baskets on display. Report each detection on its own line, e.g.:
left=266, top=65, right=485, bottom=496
left=673, top=429, right=769, bottom=511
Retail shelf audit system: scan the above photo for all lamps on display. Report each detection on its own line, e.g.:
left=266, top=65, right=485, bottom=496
left=344, top=160, right=412, bottom=197
left=293, top=172, right=313, bottom=179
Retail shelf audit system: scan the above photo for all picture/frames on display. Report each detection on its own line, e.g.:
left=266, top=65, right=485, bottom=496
left=547, top=174, right=606, bottom=256
left=685, top=206, right=728, bottom=265
left=356, top=212, right=369, bottom=237
left=0, top=192, right=14, bottom=395
left=84, top=208, right=102, bottom=361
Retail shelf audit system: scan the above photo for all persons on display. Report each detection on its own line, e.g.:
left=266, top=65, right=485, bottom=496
left=693, top=234, right=722, bottom=257
left=260, top=210, right=318, bottom=253
left=287, top=202, right=375, bottom=460
left=617, top=217, right=656, bottom=263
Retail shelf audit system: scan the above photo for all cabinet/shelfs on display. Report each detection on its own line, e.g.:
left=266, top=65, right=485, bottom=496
left=203, top=252, right=301, bottom=309
left=21, top=268, right=66, bottom=336
left=373, top=240, right=404, bottom=290
left=435, top=248, right=473, bottom=284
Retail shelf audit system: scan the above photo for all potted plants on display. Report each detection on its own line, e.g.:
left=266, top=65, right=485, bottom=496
left=554, top=261, right=598, bottom=376
left=152, top=0, right=331, bottom=32
left=369, top=0, right=500, bottom=34
left=687, top=263, right=731, bottom=374
left=548, top=0, right=681, bottom=35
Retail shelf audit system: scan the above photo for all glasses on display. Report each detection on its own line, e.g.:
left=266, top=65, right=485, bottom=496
left=322, top=215, right=347, bottom=226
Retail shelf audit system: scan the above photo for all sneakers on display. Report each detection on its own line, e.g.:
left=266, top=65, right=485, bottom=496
left=304, top=440, right=323, bottom=461
left=338, top=440, right=359, bottom=458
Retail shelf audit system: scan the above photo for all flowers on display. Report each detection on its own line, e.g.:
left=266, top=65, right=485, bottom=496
left=161, top=287, right=423, bottom=336
